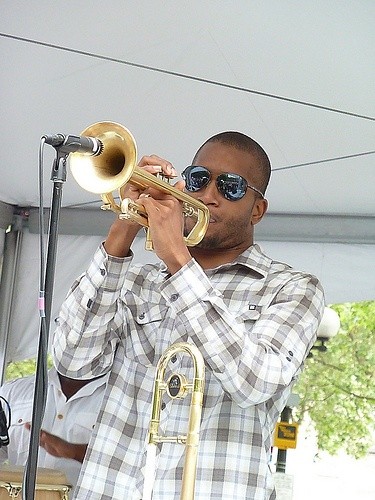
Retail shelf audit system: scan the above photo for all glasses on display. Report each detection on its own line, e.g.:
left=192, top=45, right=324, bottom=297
left=181, top=165, right=264, bottom=202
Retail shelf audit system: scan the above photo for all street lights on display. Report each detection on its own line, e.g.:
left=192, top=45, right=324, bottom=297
left=275, top=307, right=341, bottom=474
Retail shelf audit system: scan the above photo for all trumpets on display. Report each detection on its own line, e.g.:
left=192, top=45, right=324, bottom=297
left=67, top=121, right=211, bottom=257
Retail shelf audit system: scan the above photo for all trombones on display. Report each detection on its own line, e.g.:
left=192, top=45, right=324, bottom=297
left=141, top=342, right=206, bottom=500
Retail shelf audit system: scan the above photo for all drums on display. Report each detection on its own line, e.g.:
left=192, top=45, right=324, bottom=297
left=0, top=463, right=70, bottom=500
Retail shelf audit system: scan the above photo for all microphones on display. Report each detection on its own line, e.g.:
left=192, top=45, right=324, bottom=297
left=42, top=134, right=105, bottom=159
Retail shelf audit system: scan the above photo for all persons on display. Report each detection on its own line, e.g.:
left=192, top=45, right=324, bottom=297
left=53, top=131, right=325, bottom=500
left=1, top=366, right=112, bottom=500
